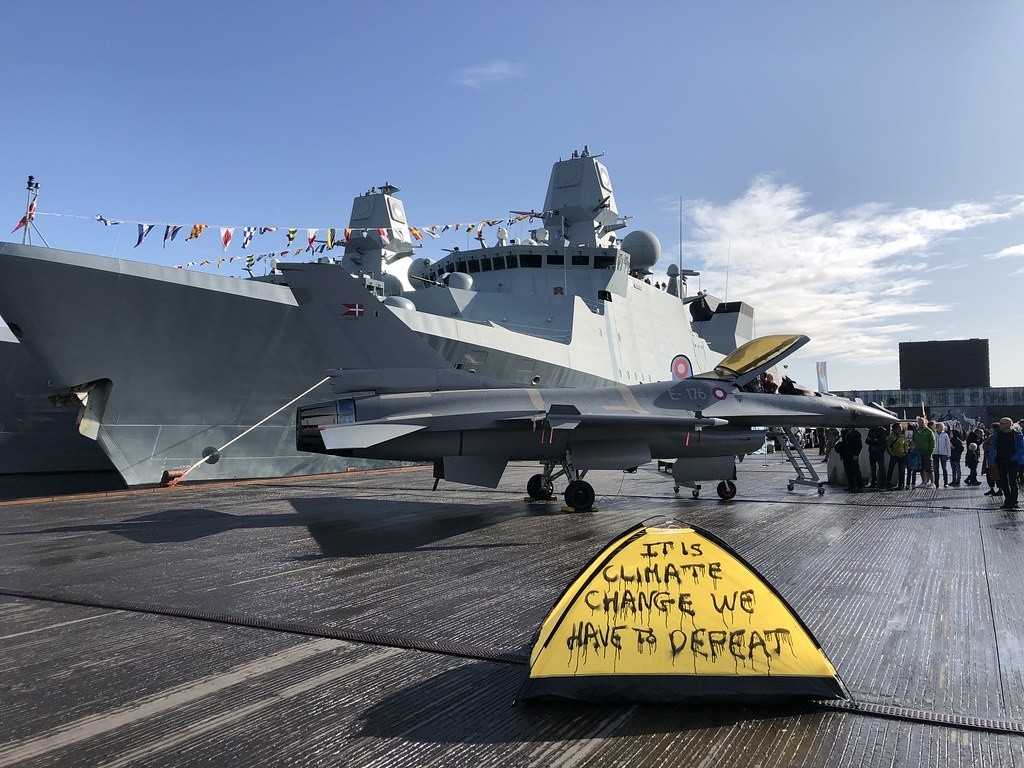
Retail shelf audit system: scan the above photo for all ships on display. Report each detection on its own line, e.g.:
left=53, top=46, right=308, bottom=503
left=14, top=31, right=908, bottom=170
left=0, top=143, right=756, bottom=487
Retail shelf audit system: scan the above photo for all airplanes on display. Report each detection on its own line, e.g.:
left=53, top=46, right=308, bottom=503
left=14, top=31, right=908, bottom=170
left=297, top=335, right=907, bottom=509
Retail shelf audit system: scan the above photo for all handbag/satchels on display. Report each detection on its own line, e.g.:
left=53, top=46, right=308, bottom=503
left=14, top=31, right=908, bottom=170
left=991, top=462, right=1001, bottom=480
left=834, top=442, right=844, bottom=459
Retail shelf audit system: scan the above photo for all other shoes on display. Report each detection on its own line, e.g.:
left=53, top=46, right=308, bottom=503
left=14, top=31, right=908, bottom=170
left=898, top=484, right=904, bottom=490
left=984, top=489, right=995, bottom=495
left=992, top=490, right=1003, bottom=496
left=906, top=485, right=909, bottom=490
left=916, top=482, right=927, bottom=488
left=844, top=487, right=863, bottom=493
left=944, top=484, right=948, bottom=487
left=927, top=483, right=936, bottom=488
left=821, top=459, right=828, bottom=462
left=912, top=485, right=916, bottom=489
left=964, top=479, right=970, bottom=484
left=948, top=482, right=960, bottom=486
left=865, top=483, right=875, bottom=488
left=819, top=452, right=827, bottom=455
left=1000, top=501, right=1020, bottom=509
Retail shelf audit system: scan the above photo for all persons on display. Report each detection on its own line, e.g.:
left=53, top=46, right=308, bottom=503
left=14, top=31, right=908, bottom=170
left=634, top=272, right=667, bottom=291
left=817, top=418, right=1024, bottom=509
left=760, top=372, right=779, bottom=393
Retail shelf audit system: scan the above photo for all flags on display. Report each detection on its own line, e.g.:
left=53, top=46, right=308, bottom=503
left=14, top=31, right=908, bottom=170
left=95, top=213, right=529, bottom=271
left=12, top=202, right=36, bottom=234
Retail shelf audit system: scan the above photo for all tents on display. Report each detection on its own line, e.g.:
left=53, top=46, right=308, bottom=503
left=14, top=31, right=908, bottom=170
left=510, top=515, right=861, bottom=714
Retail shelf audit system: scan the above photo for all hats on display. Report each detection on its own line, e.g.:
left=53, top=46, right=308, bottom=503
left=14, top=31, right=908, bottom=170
left=891, top=423, right=900, bottom=431
